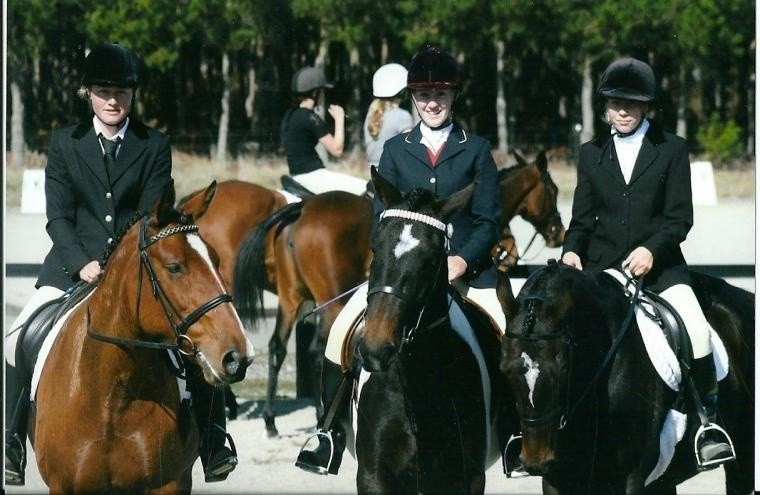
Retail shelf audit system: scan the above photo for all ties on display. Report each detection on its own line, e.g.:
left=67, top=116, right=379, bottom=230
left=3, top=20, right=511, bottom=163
left=99, top=134, right=119, bottom=177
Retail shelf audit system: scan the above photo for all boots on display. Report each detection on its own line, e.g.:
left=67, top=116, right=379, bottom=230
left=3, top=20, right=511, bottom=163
left=192, top=377, right=238, bottom=482
left=296, top=354, right=352, bottom=475
left=5, top=359, right=28, bottom=485
left=691, top=352, right=733, bottom=471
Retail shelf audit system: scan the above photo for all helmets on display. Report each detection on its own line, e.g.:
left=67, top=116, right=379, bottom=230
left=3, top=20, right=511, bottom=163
left=293, top=67, right=333, bottom=92
left=597, top=58, right=658, bottom=104
left=372, top=46, right=461, bottom=98
left=81, top=42, right=139, bottom=85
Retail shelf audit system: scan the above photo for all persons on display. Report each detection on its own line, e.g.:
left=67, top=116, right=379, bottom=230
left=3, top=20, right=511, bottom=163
left=559, top=55, right=739, bottom=468
left=361, top=61, right=415, bottom=170
left=291, top=46, right=530, bottom=477
left=5, top=41, right=238, bottom=487
left=280, top=66, right=369, bottom=197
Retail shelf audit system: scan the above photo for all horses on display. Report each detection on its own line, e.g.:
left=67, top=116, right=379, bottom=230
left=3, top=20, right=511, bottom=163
left=320, top=166, right=521, bottom=495
left=494, top=264, right=755, bottom=495
left=175, top=179, right=520, bottom=314
left=25, top=177, right=257, bottom=495
left=233, top=150, right=566, bottom=438
